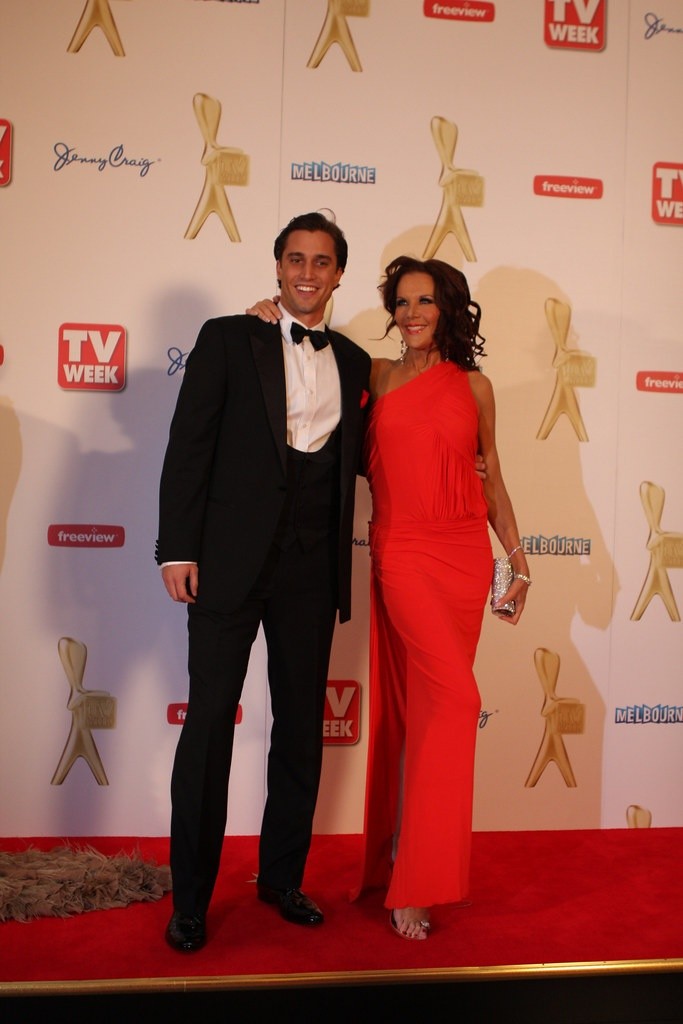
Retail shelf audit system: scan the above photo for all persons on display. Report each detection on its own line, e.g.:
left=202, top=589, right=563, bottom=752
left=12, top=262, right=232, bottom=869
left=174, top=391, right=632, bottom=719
left=154, top=212, right=489, bottom=953
left=245, top=255, right=532, bottom=941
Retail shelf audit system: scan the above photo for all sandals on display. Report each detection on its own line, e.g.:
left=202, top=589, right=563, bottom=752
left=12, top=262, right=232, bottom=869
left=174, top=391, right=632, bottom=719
left=389, top=906, right=431, bottom=941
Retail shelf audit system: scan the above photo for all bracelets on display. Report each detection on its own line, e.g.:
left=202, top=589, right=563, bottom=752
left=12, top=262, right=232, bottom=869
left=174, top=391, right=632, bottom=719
left=508, top=546, right=523, bottom=559
left=514, top=574, right=532, bottom=586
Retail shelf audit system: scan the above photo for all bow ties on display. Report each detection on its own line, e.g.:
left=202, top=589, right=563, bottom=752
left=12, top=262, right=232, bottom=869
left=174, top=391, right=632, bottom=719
left=291, top=322, right=329, bottom=351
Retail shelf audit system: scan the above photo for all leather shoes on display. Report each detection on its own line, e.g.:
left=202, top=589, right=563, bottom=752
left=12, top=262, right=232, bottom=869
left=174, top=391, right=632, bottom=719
left=258, top=884, right=324, bottom=925
left=166, top=910, right=205, bottom=952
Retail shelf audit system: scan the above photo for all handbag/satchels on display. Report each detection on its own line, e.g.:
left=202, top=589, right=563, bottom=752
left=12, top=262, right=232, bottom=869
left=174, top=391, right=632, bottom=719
left=491, top=556, right=516, bottom=617
